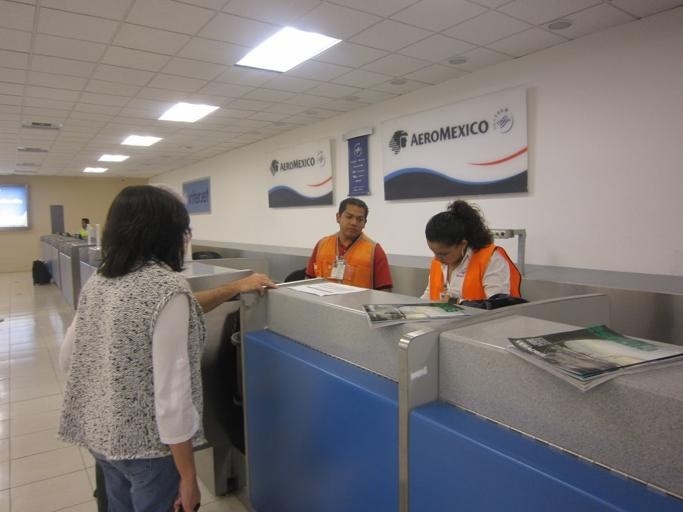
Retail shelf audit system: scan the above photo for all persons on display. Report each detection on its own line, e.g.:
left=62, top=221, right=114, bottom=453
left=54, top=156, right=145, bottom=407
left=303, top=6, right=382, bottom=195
left=302, top=195, right=395, bottom=294
left=419, top=199, right=520, bottom=306
left=78, top=217, right=89, bottom=239
left=89, top=267, right=278, bottom=512
left=51, top=183, right=209, bottom=511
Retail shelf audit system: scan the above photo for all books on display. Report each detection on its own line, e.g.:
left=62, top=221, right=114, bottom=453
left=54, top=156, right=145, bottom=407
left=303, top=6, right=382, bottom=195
left=506, top=321, right=682, bottom=393
left=361, top=298, right=471, bottom=330
